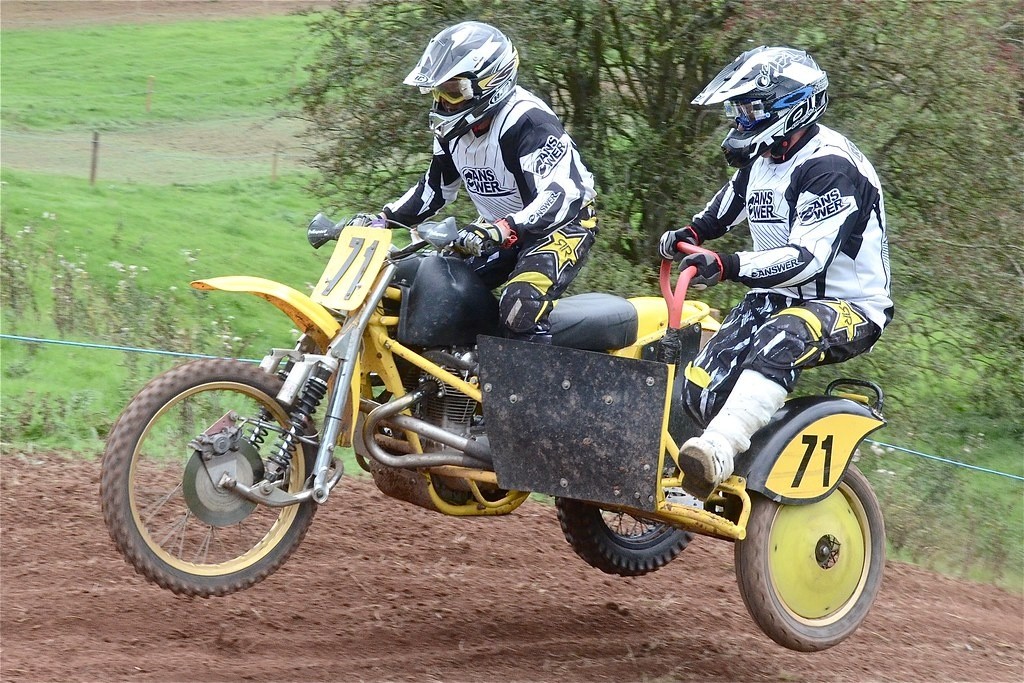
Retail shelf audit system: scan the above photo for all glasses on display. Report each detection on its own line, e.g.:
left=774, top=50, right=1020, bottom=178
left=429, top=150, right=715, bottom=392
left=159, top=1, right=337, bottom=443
left=731, top=101, right=773, bottom=122
left=418, top=75, right=476, bottom=104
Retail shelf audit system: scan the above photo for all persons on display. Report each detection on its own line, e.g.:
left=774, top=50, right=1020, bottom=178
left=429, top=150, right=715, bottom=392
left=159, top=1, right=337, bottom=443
left=656, top=43, right=893, bottom=502
left=347, top=22, right=599, bottom=344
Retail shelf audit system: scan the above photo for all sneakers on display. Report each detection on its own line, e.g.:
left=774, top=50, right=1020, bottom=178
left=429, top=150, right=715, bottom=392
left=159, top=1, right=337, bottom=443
left=677, top=436, right=724, bottom=505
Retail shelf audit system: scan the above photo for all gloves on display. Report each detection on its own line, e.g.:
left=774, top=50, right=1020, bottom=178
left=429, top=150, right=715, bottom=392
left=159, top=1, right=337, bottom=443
left=654, top=228, right=698, bottom=260
left=677, top=254, right=735, bottom=287
left=344, top=213, right=389, bottom=225
left=452, top=223, right=518, bottom=257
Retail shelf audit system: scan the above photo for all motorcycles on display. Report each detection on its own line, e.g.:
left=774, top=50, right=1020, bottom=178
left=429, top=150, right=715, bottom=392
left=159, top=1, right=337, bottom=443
left=99, top=211, right=889, bottom=654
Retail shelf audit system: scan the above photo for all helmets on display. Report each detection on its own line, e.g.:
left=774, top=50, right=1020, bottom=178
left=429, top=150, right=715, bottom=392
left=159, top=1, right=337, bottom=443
left=689, top=43, right=834, bottom=169
left=398, top=21, right=521, bottom=143
